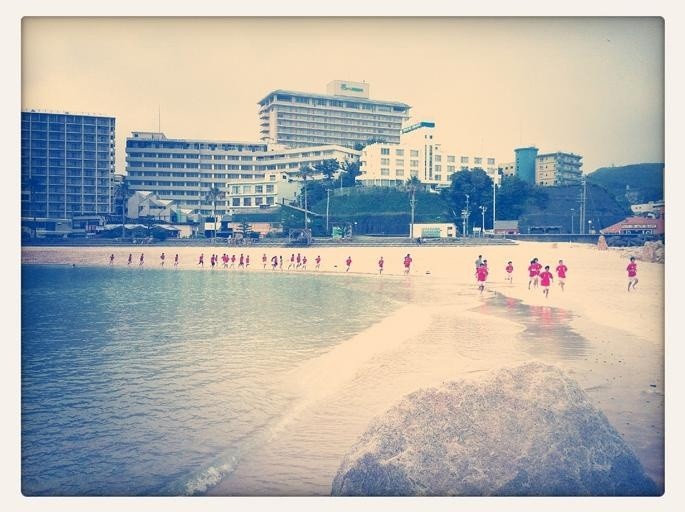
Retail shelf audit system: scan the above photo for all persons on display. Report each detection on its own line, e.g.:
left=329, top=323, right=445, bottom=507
left=159, top=252, right=166, bottom=268
left=475, top=254, right=490, bottom=296
left=108, top=254, right=115, bottom=265
left=138, top=253, right=145, bottom=266
left=378, top=256, right=385, bottom=274
left=505, top=261, right=514, bottom=284
left=127, top=254, right=134, bottom=265
left=555, top=259, right=569, bottom=293
left=527, top=260, right=538, bottom=290
left=403, top=254, right=413, bottom=276
left=539, top=265, right=555, bottom=298
left=534, top=258, right=543, bottom=286
left=345, top=256, right=353, bottom=271
left=197, top=253, right=204, bottom=267
left=210, top=252, right=322, bottom=271
left=626, top=255, right=640, bottom=292
left=173, top=254, right=180, bottom=272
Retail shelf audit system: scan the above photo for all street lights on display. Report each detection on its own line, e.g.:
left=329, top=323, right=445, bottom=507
left=570, top=207, right=575, bottom=234
left=409, top=185, right=417, bottom=223
left=578, top=180, right=586, bottom=234
left=324, top=189, right=332, bottom=230
left=479, top=204, right=489, bottom=237
left=462, top=194, right=471, bottom=237
left=588, top=221, right=592, bottom=234
left=490, top=178, right=496, bottom=222
